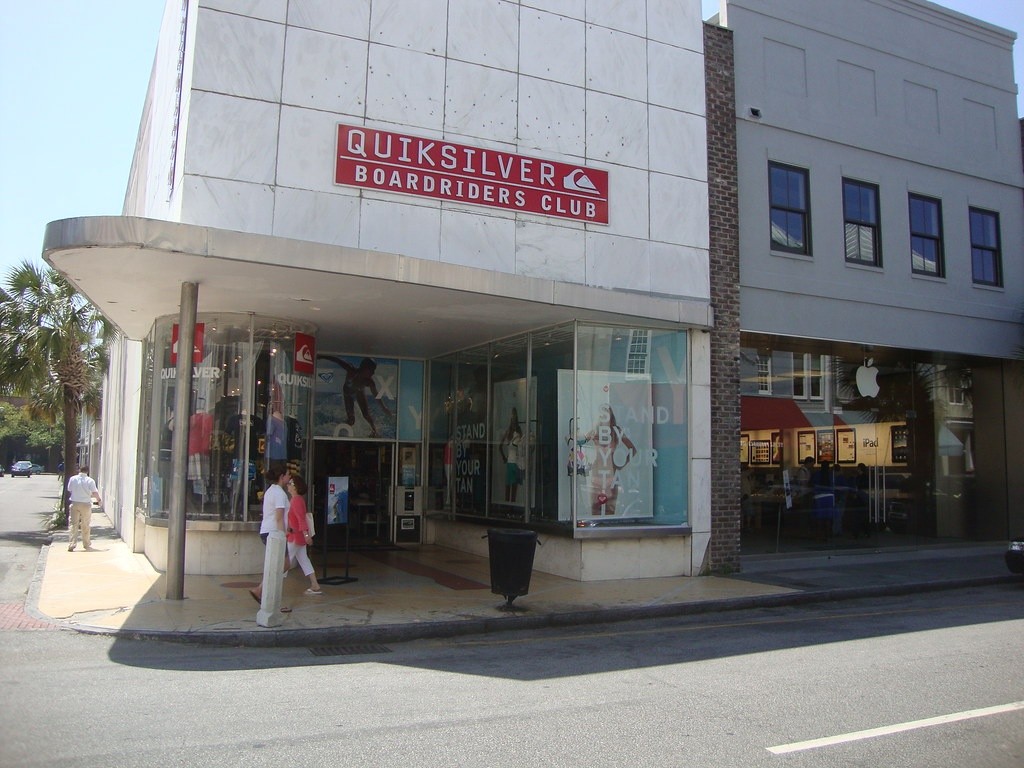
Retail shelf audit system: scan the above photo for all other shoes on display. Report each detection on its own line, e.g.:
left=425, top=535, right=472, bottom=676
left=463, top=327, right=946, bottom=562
left=84, top=542, right=92, bottom=549
left=68, top=544, right=76, bottom=552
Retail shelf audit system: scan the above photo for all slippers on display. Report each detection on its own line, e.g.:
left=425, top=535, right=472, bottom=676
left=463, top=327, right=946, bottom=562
left=281, top=606, right=292, bottom=612
left=248, top=589, right=261, bottom=605
left=303, top=588, right=322, bottom=595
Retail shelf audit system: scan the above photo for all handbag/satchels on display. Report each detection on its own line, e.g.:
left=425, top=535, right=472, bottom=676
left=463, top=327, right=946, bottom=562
left=306, top=513, right=315, bottom=537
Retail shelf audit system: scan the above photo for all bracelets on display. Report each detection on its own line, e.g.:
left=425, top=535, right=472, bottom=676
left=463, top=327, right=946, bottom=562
left=98, top=499, right=101, bottom=501
left=304, top=534, right=309, bottom=537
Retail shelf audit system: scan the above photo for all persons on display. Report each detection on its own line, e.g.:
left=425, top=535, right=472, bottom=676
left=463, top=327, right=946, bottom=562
left=157, top=408, right=213, bottom=482
left=224, top=407, right=306, bottom=477
left=317, top=354, right=395, bottom=437
left=517, top=428, right=536, bottom=475
left=444, top=437, right=456, bottom=475
left=795, top=457, right=870, bottom=546
left=499, top=407, right=523, bottom=502
left=249, top=464, right=322, bottom=614
left=565, top=426, right=590, bottom=476
left=57, top=461, right=64, bottom=483
left=67, top=466, right=103, bottom=551
left=584, top=404, right=638, bottom=516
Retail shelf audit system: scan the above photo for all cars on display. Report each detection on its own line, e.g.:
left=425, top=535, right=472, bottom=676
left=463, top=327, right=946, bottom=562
left=879, top=477, right=987, bottom=538
left=774, top=485, right=885, bottom=539
left=31, top=463, right=43, bottom=475
left=0, top=464, right=5, bottom=477
left=11, top=461, right=33, bottom=478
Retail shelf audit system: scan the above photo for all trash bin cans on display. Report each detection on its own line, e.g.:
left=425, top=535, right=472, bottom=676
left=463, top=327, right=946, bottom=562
left=485, top=528, right=538, bottom=598
